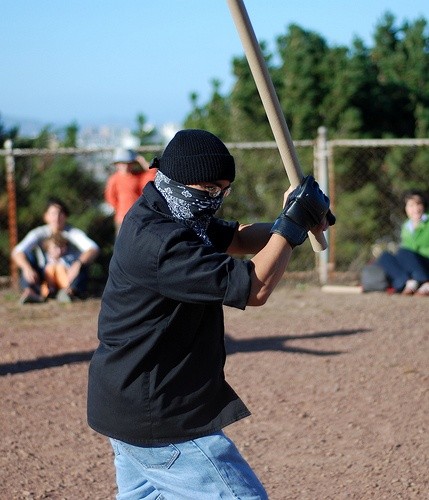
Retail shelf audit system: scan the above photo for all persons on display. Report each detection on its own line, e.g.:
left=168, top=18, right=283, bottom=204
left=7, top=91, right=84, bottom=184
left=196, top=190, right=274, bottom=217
left=369, top=190, right=429, bottom=299
left=9, top=195, right=101, bottom=305
left=84, top=128, right=337, bottom=500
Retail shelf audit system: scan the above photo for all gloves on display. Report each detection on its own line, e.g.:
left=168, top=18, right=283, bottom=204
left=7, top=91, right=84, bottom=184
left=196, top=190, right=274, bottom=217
left=270, top=175, right=336, bottom=251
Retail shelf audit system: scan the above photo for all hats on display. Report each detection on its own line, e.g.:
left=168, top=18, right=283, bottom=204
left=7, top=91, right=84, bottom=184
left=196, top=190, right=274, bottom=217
left=158, top=129, right=235, bottom=185
left=109, top=149, right=139, bottom=165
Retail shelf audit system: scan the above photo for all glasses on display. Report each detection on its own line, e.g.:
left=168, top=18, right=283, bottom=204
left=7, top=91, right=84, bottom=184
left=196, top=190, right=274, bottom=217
left=203, top=184, right=232, bottom=198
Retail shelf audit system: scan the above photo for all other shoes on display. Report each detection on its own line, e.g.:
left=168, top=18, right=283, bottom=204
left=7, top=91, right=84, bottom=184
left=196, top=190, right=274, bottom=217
left=57, top=289, right=78, bottom=303
left=402, top=280, right=418, bottom=296
left=412, top=282, right=429, bottom=296
left=20, top=294, right=44, bottom=304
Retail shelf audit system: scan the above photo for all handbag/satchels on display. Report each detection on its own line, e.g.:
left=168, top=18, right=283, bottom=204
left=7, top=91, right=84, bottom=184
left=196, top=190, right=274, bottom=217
left=360, top=265, right=389, bottom=291
